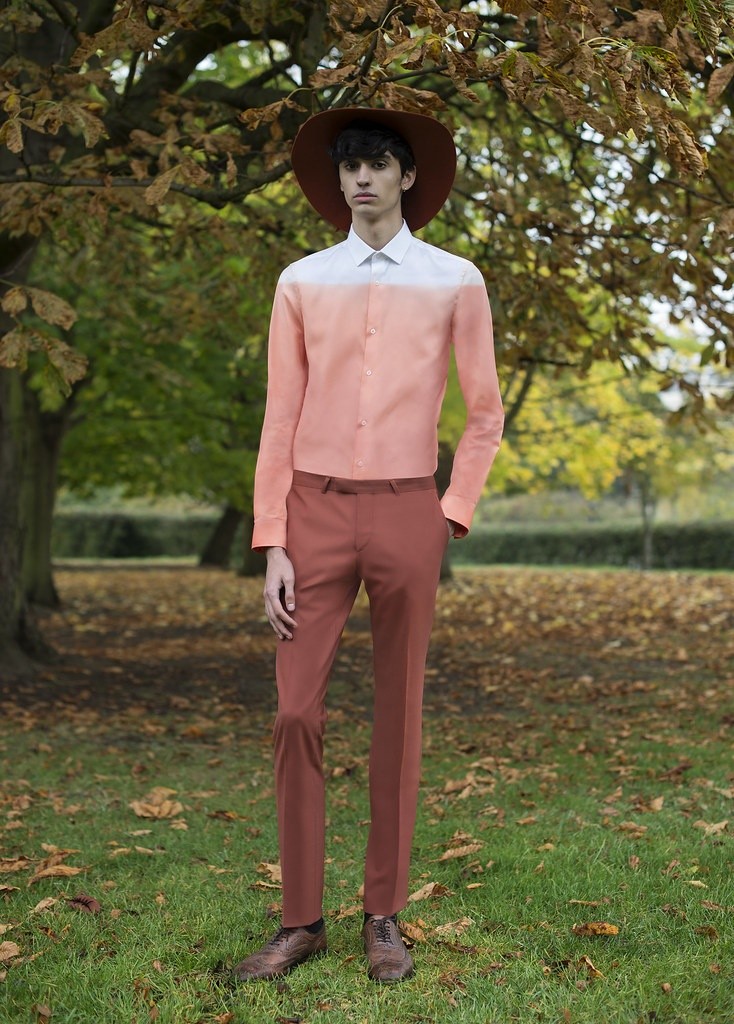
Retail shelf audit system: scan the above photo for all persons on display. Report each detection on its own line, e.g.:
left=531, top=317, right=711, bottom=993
left=231, top=107, right=505, bottom=988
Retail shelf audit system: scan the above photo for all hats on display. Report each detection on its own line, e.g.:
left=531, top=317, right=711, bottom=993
left=291, top=108, right=457, bottom=233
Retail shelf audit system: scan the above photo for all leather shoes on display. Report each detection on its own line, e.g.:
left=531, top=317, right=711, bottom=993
left=233, top=918, right=328, bottom=980
left=360, top=914, right=413, bottom=983
